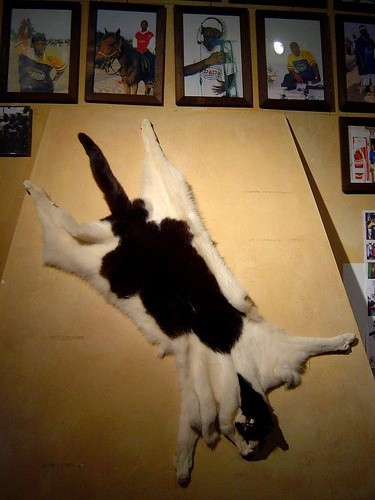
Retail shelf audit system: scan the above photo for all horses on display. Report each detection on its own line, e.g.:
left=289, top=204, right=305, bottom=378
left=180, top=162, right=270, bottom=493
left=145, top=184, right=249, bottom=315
left=93, top=26, right=155, bottom=96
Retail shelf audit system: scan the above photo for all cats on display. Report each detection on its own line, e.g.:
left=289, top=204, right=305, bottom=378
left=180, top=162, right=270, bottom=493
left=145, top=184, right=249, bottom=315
left=22, top=116, right=356, bottom=481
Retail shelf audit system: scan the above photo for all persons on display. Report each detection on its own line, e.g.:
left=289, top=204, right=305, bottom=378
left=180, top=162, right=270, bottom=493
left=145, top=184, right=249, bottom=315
left=369, top=243, right=375, bottom=259
left=369, top=144, right=375, bottom=165
left=366, top=215, right=375, bottom=240
left=0, top=113, right=29, bottom=150
left=280, top=42, right=322, bottom=100
left=115, top=20, right=155, bottom=87
left=14, top=17, right=67, bottom=93
left=354, top=25, right=375, bottom=97
left=184, top=17, right=237, bottom=97
